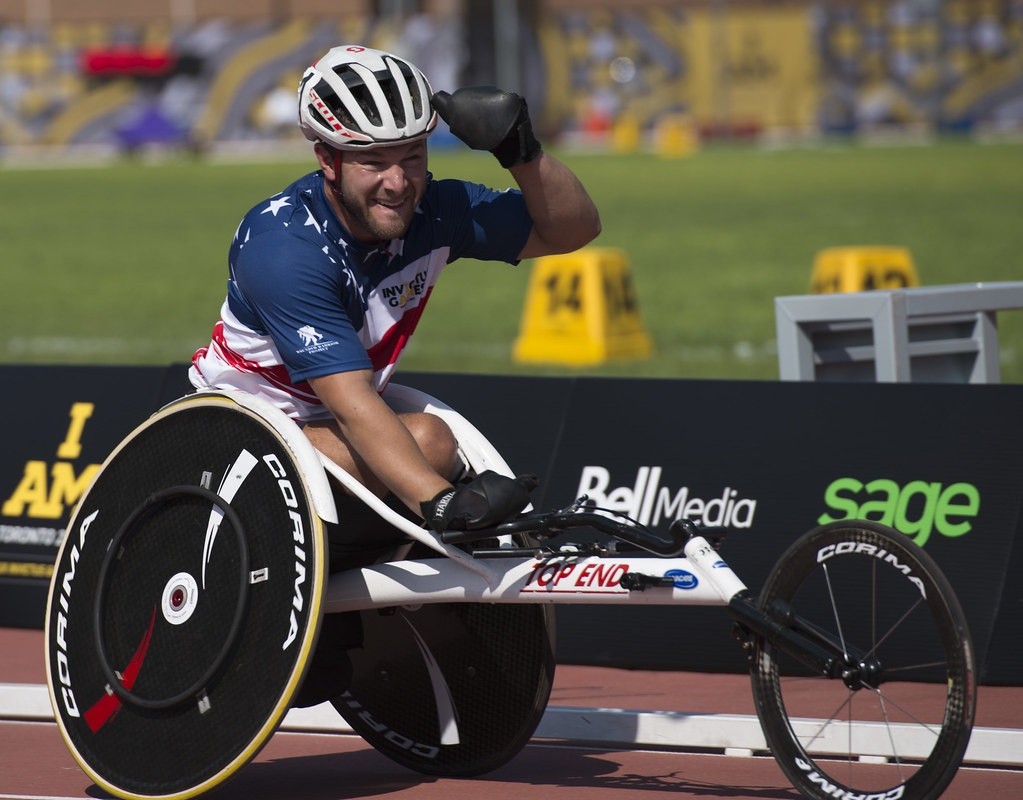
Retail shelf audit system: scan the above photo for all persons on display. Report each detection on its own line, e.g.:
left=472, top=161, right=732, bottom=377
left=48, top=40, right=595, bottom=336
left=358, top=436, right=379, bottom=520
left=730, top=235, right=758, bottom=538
left=178, top=42, right=601, bottom=543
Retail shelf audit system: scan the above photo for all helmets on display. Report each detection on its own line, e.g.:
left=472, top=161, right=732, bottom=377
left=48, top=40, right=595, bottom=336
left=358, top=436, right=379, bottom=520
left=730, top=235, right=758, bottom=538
left=296, top=44, right=438, bottom=150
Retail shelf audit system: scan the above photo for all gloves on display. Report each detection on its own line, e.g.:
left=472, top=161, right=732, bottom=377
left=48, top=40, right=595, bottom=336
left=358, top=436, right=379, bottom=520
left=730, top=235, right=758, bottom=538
left=427, top=470, right=540, bottom=532
left=432, top=85, right=541, bottom=168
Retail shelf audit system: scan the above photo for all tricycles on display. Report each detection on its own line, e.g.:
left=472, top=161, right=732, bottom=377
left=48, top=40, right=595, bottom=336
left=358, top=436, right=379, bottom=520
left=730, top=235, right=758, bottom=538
left=45, top=382, right=976, bottom=799
